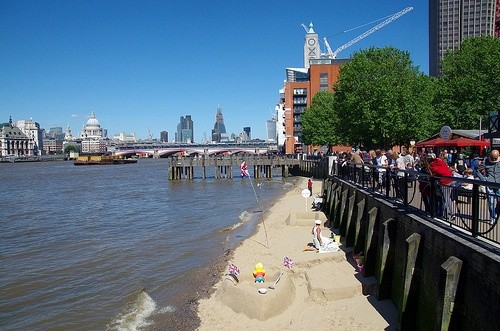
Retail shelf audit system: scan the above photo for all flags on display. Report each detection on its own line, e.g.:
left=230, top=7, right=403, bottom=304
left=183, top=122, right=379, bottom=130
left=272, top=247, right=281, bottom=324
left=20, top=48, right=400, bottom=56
left=240, top=161, right=250, bottom=178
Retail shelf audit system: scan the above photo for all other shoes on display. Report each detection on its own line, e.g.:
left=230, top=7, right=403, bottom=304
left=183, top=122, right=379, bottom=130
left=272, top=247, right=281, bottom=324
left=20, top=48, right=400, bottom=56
left=490, top=215, right=497, bottom=224
left=305, top=246, right=307, bottom=250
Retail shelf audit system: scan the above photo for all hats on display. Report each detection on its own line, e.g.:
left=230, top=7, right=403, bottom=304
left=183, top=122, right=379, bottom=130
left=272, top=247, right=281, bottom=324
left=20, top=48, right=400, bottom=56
left=315, top=220, right=321, bottom=224
left=426, top=154, right=435, bottom=159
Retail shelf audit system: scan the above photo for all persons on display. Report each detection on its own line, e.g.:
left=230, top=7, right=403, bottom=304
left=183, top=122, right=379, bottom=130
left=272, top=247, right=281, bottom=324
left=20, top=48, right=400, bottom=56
left=312, top=147, right=500, bottom=227
left=311, top=220, right=327, bottom=249
left=87, top=154, right=91, bottom=161
left=308, top=179, right=313, bottom=197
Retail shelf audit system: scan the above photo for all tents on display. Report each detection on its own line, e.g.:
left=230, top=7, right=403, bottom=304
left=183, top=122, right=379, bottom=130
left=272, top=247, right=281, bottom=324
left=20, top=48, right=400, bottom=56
left=419, top=137, right=490, bottom=156
